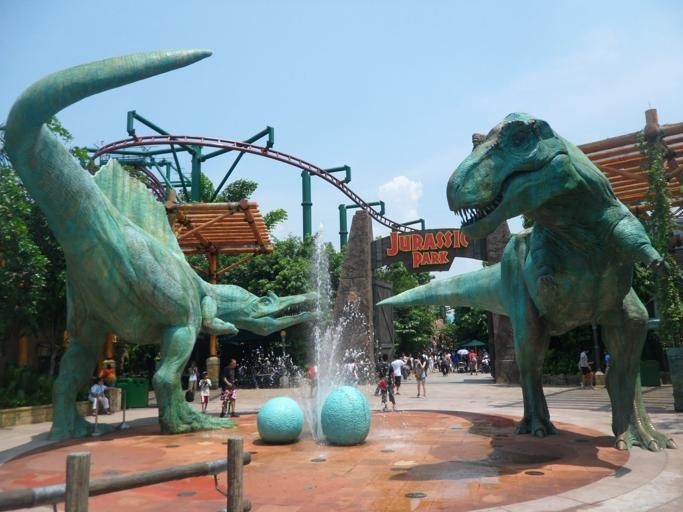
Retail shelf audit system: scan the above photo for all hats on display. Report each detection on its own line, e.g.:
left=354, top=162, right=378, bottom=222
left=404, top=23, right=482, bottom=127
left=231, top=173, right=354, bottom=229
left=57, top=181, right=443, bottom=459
left=202, top=371, right=208, bottom=375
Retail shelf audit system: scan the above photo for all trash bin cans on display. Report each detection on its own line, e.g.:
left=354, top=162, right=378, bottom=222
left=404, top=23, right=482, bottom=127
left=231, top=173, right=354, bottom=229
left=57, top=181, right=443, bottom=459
left=641, top=360, right=660, bottom=386
left=116, top=377, right=149, bottom=408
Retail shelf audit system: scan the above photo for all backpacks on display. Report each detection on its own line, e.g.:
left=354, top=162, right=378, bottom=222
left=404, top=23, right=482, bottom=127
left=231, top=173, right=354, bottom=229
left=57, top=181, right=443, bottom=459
left=184, top=390, right=195, bottom=402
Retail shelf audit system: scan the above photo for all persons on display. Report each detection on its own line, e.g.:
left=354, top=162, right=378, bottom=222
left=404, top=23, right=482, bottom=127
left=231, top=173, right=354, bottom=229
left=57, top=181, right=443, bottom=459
left=188, top=357, right=359, bottom=418
left=576, top=352, right=594, bottom=388
left=375, top=348, right=491, bottom=412
left=96, top=364, right=117, bottom=386
left=579, top=347, right=597, bottom=390
left=87, top=376, right=117, bottom=416
left=601, top=349, right=612, bottom=389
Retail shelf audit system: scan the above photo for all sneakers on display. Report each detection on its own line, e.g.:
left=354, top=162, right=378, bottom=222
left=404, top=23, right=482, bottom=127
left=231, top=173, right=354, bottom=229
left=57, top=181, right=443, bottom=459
left=580, top=383, right=595, bottom=389
left=89, top=409, right=110, bottom=415
left=220, top=412, right=240, bottom=417
left=381, top=392, right=427, bottom=413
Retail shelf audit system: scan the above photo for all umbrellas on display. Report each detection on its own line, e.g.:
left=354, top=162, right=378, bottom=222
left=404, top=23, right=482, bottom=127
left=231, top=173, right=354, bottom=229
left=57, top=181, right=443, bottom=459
left=461, top=338, right=486, bottom=354
